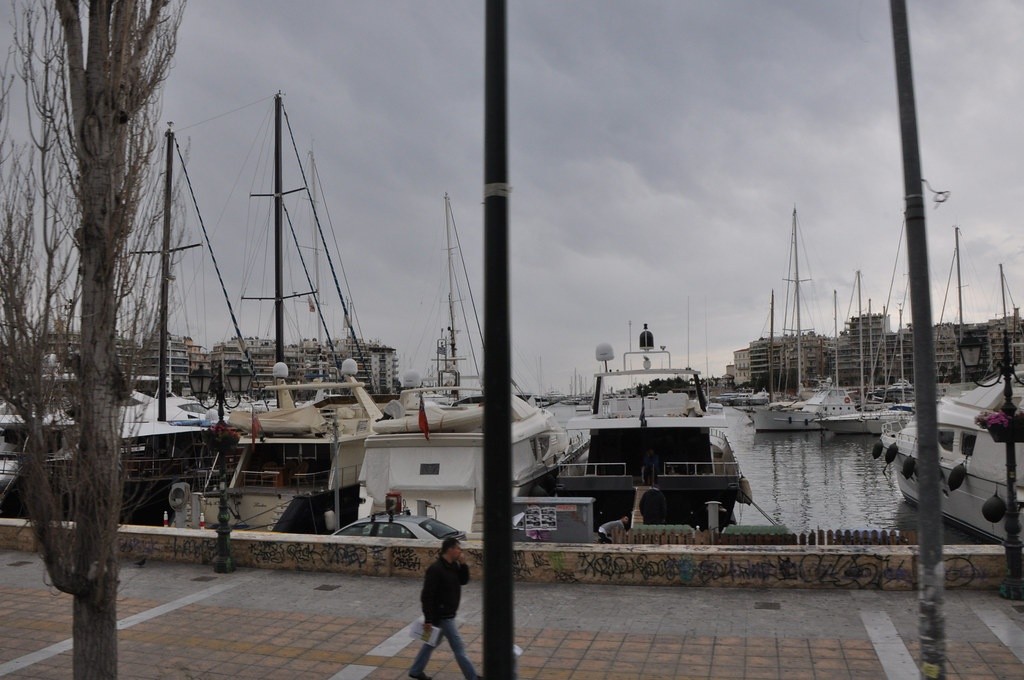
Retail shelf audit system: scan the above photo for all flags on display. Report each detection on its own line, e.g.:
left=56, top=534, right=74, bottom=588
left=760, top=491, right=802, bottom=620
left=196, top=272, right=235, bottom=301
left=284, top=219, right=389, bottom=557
left=419, top=396, right=429, bottom=440
left=251, top=410, right=265, bottom=443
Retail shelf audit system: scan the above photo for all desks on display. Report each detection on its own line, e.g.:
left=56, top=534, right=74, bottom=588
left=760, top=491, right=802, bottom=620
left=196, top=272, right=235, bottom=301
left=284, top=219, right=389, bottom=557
left=263, top=466, right=285, bottom=487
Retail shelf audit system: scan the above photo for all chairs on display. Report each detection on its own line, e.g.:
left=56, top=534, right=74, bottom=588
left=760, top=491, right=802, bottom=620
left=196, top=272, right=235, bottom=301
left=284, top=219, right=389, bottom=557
left=261, top=462, right=278, bottom=486
left=288, top=463, right=310, bottom=488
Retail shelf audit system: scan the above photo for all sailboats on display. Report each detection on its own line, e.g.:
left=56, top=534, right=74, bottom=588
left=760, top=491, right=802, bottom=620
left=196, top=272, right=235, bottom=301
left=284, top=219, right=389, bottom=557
left=560, top=367, right=579, bottom=405
left=1, top=90, right=1024, bottom=547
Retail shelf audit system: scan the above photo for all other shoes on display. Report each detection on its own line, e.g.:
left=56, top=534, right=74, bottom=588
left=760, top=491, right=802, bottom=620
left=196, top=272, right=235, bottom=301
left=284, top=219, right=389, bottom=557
left=409, top=671, right=432, bottom=680
left=465, top=674, right=485, bottom=680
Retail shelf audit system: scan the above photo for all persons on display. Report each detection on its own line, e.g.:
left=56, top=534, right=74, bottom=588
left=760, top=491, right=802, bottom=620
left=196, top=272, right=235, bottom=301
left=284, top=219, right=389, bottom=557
left=408, top=538, right=481, bottom=680
left=640, top=485, right=666, bottom=525
left=597, top=515, right=629, bottom=542
left=643, top=448, right=657, bottom=483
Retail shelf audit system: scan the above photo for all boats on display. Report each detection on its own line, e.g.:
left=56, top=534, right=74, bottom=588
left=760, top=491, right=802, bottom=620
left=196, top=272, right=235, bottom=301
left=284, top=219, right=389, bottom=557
left=30, top=421, right=239, bottom=516
left=0, top=413, right=76, bottom=519
left=733, top=389, right=856, bottom=433
left=747, top=392, right=768, bottom=405
left=730, top=394, right=750, bottom=405
left=358, top=327, right=590, bottom=536
left=711, top=393, right=739, bottom=406
left=170, top=358, right=384, bottom=532
left=815, top=401, right=943, bottom=435
left=513, top=320, right=753, bottom=533
left=872, top=372, right=1024, bottom=545
